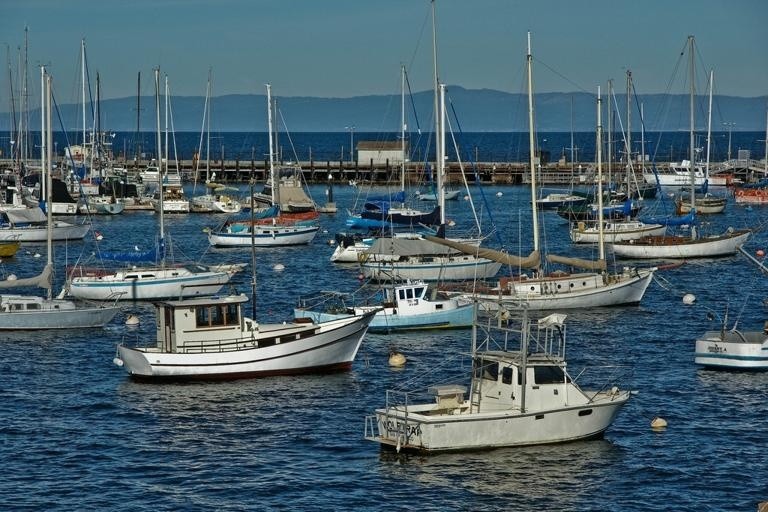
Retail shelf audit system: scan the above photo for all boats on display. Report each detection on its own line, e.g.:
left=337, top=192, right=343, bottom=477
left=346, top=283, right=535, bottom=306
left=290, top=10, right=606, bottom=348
left=351, top=293, right=635, bottom=454
